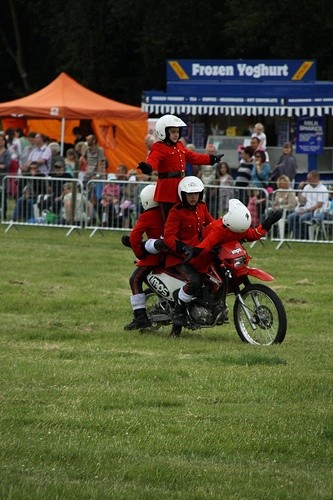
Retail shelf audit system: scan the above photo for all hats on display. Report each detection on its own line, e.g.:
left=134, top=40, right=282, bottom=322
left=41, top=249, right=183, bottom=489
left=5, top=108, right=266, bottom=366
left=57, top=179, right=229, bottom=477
left=54, top=161, right=64, bottom=167
left=107, top=173, right=117, bottom=180
left=129, top=176, right=137, bottom=181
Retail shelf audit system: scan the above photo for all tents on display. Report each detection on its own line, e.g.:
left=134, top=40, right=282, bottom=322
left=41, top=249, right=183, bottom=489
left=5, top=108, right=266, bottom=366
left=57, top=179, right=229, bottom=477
left=0, top=72, right=149, bottom=174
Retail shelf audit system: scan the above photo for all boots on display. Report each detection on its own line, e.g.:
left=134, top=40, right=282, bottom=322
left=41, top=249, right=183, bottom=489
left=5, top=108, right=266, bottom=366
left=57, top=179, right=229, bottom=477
left=123, top=292, right=152, bottom=331
left=121, top=235, right=159, bottom=256
left=168, top=286, right=194, bottom=337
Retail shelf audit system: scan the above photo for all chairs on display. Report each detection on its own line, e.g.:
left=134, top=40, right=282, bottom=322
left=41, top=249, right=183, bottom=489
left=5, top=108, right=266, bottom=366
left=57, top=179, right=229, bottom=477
left=270, top=196, right=333, bottom=241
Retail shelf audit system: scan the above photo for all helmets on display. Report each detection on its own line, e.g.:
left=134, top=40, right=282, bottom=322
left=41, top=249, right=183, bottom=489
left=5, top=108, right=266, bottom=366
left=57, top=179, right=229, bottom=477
left=155, top=115, right=187, bottom=141
left=178, top=176, right=204, bottom=202
left=221, top=199, right=252, bottom=233
left=138, top=185, right=160, bottom=210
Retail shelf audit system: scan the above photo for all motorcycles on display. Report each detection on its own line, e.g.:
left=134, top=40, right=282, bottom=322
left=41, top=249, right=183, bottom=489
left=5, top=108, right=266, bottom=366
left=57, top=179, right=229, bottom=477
left=142, top=237, right=288, bottom=346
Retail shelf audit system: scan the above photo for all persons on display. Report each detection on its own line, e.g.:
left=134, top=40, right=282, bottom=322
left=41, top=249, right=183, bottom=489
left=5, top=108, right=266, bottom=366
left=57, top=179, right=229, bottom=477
left=0, top=126, right=157, bottom=229
left=178, top=123, right=333, bottom=240
left=145, top=114, right=224, bottom=241
left=124, top=176, right=284, bottom=338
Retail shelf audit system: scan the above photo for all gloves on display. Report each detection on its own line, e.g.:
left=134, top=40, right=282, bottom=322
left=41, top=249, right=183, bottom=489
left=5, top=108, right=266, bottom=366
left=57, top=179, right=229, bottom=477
left=213, top=154, right=225, bottom=164
left=136, top=161, right=152, bottom=175
left=262, top=210, right=283, bottom=232
left=183, top=249, right=195, bottom=265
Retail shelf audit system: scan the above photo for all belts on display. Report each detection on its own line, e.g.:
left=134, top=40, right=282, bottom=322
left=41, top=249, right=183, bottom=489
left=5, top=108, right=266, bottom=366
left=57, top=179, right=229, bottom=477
left=158, top=171, right=183, bottom=178
